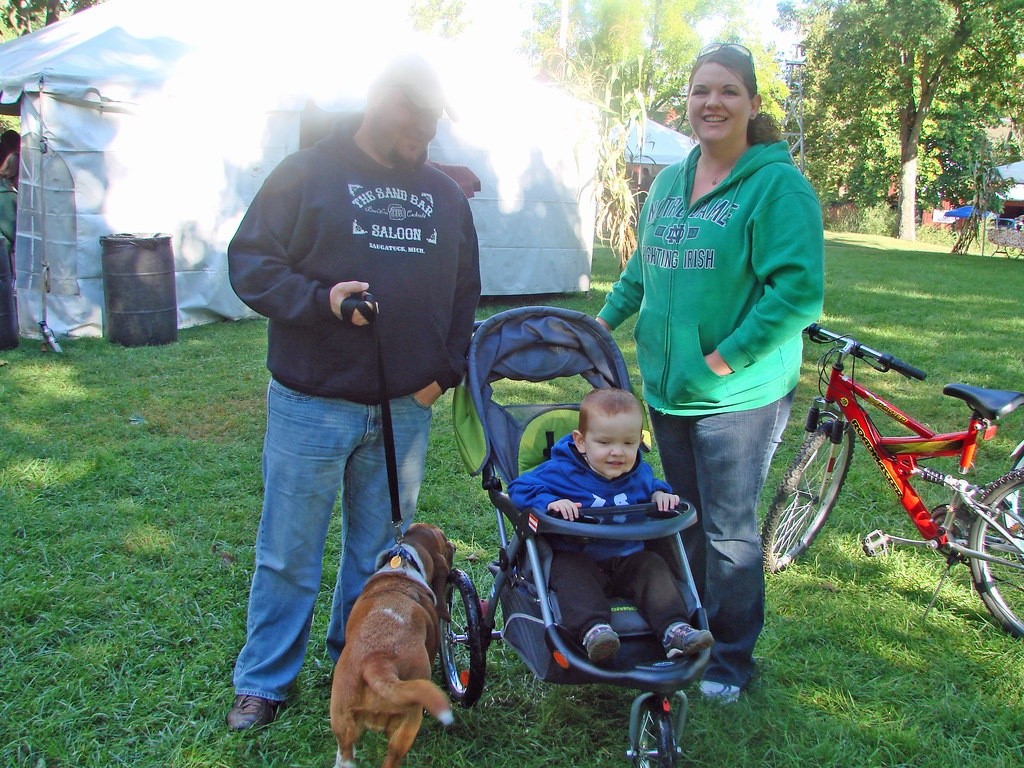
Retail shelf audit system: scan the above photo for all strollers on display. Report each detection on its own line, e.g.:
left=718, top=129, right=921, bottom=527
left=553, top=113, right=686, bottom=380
left=439, top=306, right=713, bottom=768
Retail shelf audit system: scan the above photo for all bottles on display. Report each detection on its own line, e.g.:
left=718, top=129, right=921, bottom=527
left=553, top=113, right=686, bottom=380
left=38, top=320, right=63, bottom=352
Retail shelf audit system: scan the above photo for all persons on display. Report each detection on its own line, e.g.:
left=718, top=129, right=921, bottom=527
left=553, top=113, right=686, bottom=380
left=597, top=48, right=825, bottom=704
left=0, top=130, right=20, bottom=349
left=225, top=62, right=481, bottom=733
left=641, top=168, right=654, bottom=191
left=508, top=388, right=715, bottom=662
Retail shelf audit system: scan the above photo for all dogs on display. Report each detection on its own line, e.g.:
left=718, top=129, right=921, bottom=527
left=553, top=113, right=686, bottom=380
left=328, top=521, right=458, bottom=766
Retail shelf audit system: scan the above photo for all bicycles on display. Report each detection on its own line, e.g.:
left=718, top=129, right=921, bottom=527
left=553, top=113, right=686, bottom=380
left=760, top=324, right=1024, bottom=638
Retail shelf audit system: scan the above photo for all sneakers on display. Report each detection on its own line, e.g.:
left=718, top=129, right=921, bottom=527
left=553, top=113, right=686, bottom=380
left=227, top=686, right=279, bottom=740
left=700, top=674, right=739, bottom=706
left=661, top=621, right=712, bottom=660
left=583, top=623, right=622, bottom=664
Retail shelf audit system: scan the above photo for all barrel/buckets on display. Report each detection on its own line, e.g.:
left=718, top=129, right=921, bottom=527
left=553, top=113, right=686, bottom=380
left=99, top=232, right=178, bottom=347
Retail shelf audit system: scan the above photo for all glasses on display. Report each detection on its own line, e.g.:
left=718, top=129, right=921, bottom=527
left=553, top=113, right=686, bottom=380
left=695, top=40, right=754, bottom=64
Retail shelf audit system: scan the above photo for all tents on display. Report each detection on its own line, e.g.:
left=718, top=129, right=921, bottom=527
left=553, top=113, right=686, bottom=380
left=624, top=116, right=697, bottom=164
left=0, top=1, right=594, bottom=339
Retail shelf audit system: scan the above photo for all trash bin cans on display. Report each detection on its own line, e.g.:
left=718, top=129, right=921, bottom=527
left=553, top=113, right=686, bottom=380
left=98, top=232, right=178, bottom=346
left=0, top=239, right=20, bottom=350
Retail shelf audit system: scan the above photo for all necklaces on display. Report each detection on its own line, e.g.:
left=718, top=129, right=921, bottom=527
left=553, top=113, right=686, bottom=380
left=700, top=156, right=716, bottom=185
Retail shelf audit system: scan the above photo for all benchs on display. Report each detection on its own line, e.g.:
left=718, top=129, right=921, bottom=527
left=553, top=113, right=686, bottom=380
left=988, top=229, right=1024, bottom=260
